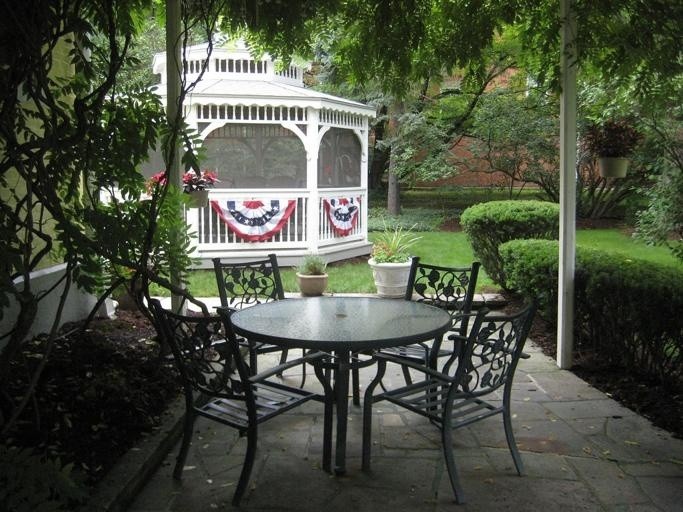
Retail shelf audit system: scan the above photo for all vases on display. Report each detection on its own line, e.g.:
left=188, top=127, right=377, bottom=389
left=187, top=190, right=208, bottom=208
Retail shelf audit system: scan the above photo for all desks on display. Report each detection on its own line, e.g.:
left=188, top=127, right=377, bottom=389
left=229, top=297, right=451, bottom=474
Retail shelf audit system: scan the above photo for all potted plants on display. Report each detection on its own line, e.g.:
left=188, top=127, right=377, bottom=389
left=367, top=213, right=425, bottom=299
left=586, top=118, right=643, bottom=179
left=293, top=253, right=329, bottom=297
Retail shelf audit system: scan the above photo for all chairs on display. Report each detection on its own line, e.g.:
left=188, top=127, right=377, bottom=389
left=352, top=258, right=482, bottom=416
left=149, top=299, right=337, bottom=508
left=212, top=253, right=334, bottom=396
left=362, top=296, right=537, bottom=504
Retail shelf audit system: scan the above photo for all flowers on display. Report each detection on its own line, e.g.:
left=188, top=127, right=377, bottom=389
left=183, top=170, right=222, bottom=190
